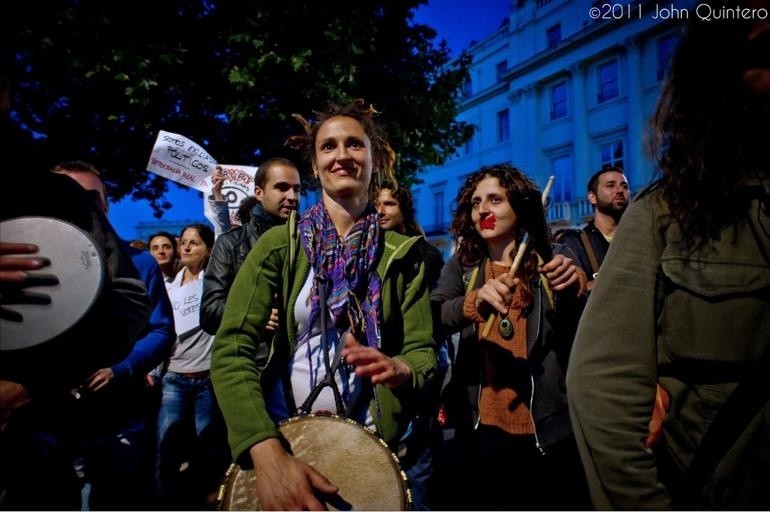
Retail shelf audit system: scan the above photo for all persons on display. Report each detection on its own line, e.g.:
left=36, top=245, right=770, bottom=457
left=563, top=0, right=770, bottom=510
left=0, top=97, right=671, bottom=511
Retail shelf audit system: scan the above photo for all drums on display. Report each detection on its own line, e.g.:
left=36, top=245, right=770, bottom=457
left=214, top=410, right=414, bottom=511
left=1, top=217, right=107, bottom=351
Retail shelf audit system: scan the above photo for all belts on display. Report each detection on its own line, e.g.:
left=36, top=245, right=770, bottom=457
left=179, top=371, right=211, bottom=379
